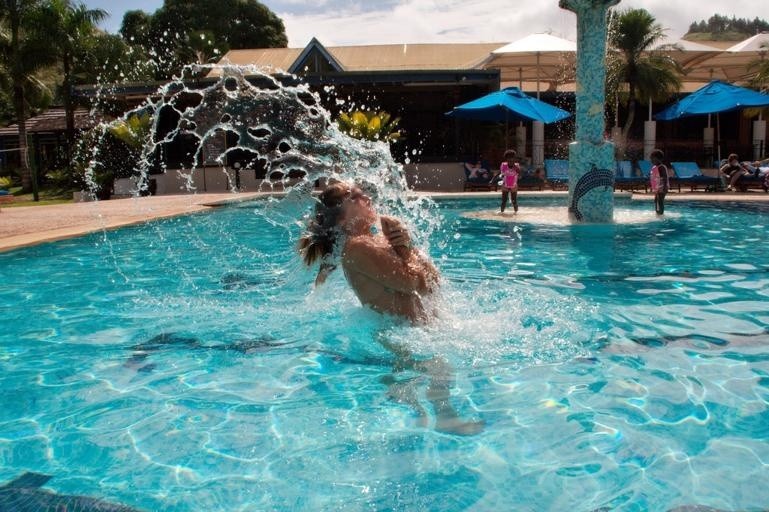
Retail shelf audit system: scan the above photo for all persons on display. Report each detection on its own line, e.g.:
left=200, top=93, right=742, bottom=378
left=496, top=149, right=520, bottom=216
left=297, top=182, right=486, bottom=437
left=465, top=160, right=487, bottom=179
left=649, top=150, right=670, bottom=217
left=720, top=153, right=769, bottom=192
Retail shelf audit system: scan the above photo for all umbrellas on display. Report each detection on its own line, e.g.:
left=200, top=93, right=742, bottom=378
left=653, top=79, right=769, bottom=182
left=443, top=86, right=573, bottom=154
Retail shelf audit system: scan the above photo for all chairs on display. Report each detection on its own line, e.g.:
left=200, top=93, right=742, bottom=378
left=460, top=158, right=769, bottom=192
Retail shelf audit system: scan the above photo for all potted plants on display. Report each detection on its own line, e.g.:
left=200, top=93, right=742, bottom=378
left=110, top=112, right=157, bottom=196
